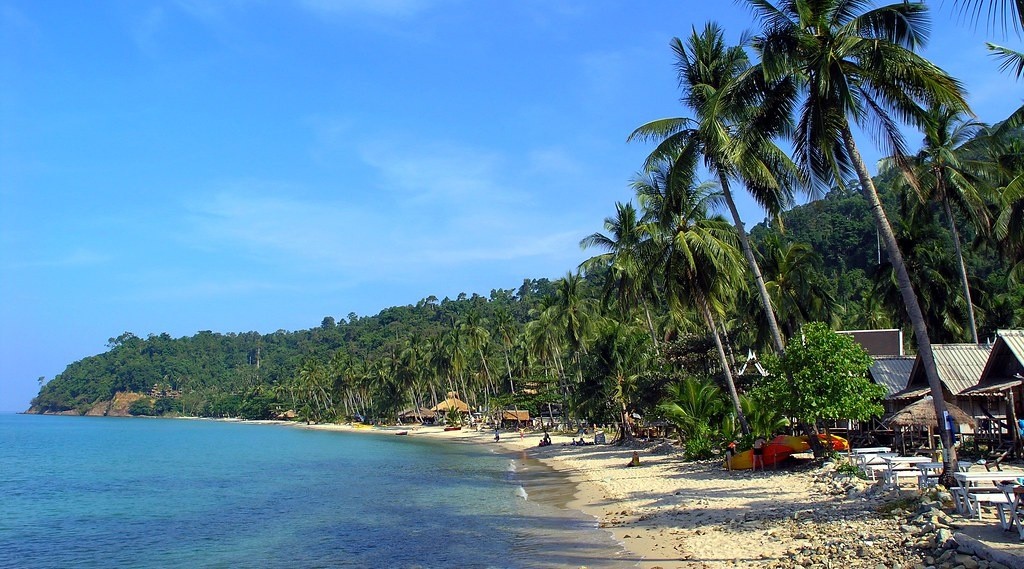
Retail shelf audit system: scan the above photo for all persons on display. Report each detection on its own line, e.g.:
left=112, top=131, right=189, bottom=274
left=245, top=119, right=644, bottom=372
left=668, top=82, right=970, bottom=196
left=494, top=430, right=499, bottom=442
left=570, top=438, right=584, bottom=445
left=725, top=440, right=741, bottom=474
left=539, top=431, right=551, bottom=447
left=752, top=437, right=765, bottom=471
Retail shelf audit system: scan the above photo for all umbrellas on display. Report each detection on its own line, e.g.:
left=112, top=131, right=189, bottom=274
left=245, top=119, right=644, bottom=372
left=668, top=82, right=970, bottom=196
left=888, top=394, right=976, bottom=450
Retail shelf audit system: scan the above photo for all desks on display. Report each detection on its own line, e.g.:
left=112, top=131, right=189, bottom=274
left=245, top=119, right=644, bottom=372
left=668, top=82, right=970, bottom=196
left=852, top=448, right=890, bottom=467
left=885, top=456, right=932, bottom=479
left=955, top=472, right=1023, bottom=502
left=917, top=461, right=970, bottom=480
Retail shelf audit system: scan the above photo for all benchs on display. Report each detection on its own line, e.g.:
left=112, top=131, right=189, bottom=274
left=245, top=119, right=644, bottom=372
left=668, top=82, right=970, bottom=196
left=837, top=454, right=1024, bottom=540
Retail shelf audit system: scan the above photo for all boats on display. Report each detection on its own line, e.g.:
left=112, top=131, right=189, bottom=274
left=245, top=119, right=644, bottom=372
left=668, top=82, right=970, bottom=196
left=395, top=431, right=408, bottom=436
